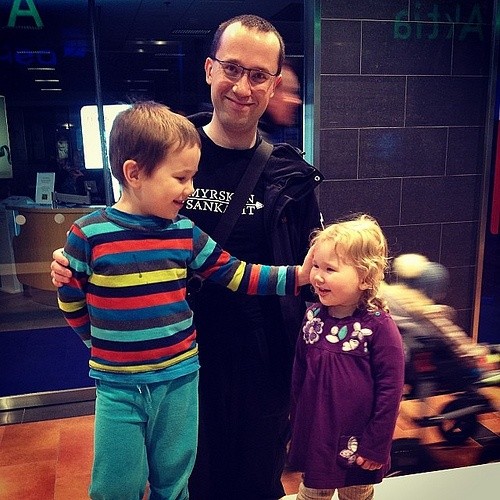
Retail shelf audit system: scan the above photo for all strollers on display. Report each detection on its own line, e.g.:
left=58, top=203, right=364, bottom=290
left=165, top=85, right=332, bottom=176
left=380, top=280, right=500, bottom=474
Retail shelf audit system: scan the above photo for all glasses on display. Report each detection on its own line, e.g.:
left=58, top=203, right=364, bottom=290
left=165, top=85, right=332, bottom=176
left=212, top=57, right=280, bottom=85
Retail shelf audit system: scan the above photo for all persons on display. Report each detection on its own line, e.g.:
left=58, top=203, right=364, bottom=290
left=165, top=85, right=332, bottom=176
left=57, top=100, right=315, bottom=500
left=50, top=14, right=304, bottom=500
left=286, top=214, right=405, bottom=500
